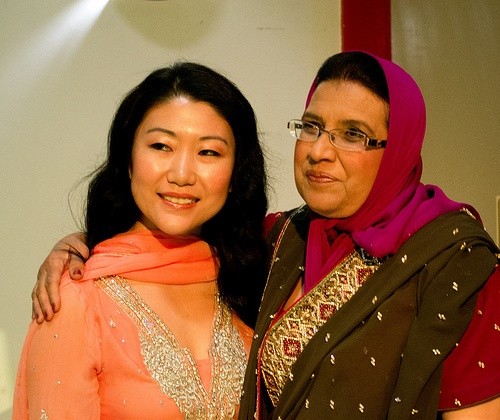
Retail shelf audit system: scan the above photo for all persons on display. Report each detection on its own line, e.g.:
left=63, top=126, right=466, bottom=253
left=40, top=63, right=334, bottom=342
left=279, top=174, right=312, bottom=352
left=13, top=62, right=271, bottom=420
left=29, top=50, right=500, bottom=420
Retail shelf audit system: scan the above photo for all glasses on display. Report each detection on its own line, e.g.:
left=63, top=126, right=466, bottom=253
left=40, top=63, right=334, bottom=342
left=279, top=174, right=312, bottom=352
left=288, top=119, right=387, bottom=152
